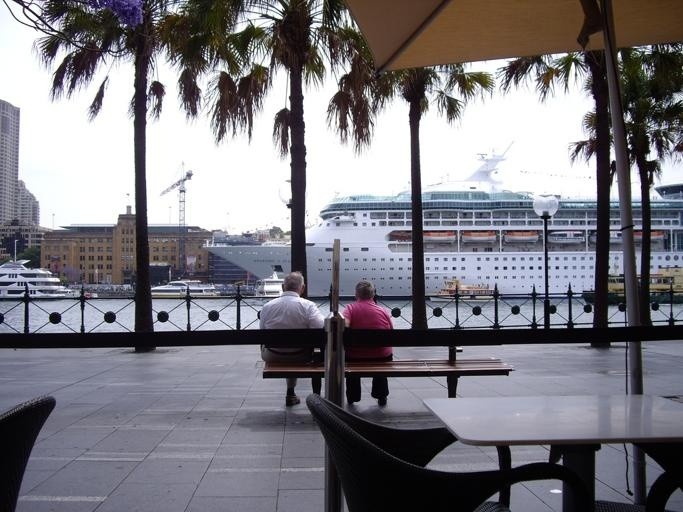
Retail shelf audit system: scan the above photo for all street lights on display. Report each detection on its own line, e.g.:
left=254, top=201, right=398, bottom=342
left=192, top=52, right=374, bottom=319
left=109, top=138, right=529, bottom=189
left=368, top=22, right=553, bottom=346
left=530, top=188, right=560, bottom=344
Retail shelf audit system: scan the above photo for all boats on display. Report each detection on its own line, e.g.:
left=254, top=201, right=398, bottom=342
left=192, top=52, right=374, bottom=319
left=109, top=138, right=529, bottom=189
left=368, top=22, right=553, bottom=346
left=428, top=275, right=504, bottom=307
left=150, top=275, right=223, bottom=300
left=581, top=261, right=683, bottom=305
left=0, top=257, right=83, bottom=300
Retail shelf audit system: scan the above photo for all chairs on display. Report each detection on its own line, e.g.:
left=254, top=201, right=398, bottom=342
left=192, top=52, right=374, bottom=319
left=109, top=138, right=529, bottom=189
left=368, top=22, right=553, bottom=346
left=595, top=395, right=683, bottom=512
left=306, top=394, right=589, bottom=512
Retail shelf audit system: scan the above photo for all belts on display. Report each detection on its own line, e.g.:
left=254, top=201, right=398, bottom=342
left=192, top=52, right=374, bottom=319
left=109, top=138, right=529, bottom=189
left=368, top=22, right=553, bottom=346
left=266, top=346, right=307, bottom=357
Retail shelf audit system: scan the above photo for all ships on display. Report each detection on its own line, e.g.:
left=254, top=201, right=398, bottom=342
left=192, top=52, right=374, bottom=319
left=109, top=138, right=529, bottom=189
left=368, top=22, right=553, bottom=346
left=198, top=174, right=683, bottom=303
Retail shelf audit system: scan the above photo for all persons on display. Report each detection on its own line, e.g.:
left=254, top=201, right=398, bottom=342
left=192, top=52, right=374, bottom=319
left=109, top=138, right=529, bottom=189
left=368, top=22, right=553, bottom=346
left=341, top=280, right=394, bottom=408
left=259, top=269, right=325, bottom=406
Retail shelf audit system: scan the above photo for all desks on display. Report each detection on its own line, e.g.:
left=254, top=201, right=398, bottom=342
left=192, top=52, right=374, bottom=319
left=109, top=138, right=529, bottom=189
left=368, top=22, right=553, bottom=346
left=422, top=394, right=683, bottom=512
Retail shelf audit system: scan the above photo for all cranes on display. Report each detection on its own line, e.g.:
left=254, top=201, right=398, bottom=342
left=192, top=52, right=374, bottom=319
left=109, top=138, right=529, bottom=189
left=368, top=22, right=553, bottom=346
left=159, top=163, right=194, bottom=281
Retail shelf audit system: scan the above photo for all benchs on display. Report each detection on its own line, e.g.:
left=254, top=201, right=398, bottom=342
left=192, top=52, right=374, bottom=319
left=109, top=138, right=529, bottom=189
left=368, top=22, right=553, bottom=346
left=263, top=358, right=516, bottom=397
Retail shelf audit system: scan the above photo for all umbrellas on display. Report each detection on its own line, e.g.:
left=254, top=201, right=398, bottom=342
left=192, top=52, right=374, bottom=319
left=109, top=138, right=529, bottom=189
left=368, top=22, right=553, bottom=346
left=342, top=0, right=682, bottom=503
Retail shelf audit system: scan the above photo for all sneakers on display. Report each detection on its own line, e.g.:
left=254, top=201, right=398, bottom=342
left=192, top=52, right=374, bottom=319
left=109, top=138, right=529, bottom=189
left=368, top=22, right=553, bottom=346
left=285, top=393, right=388, bottom=406
